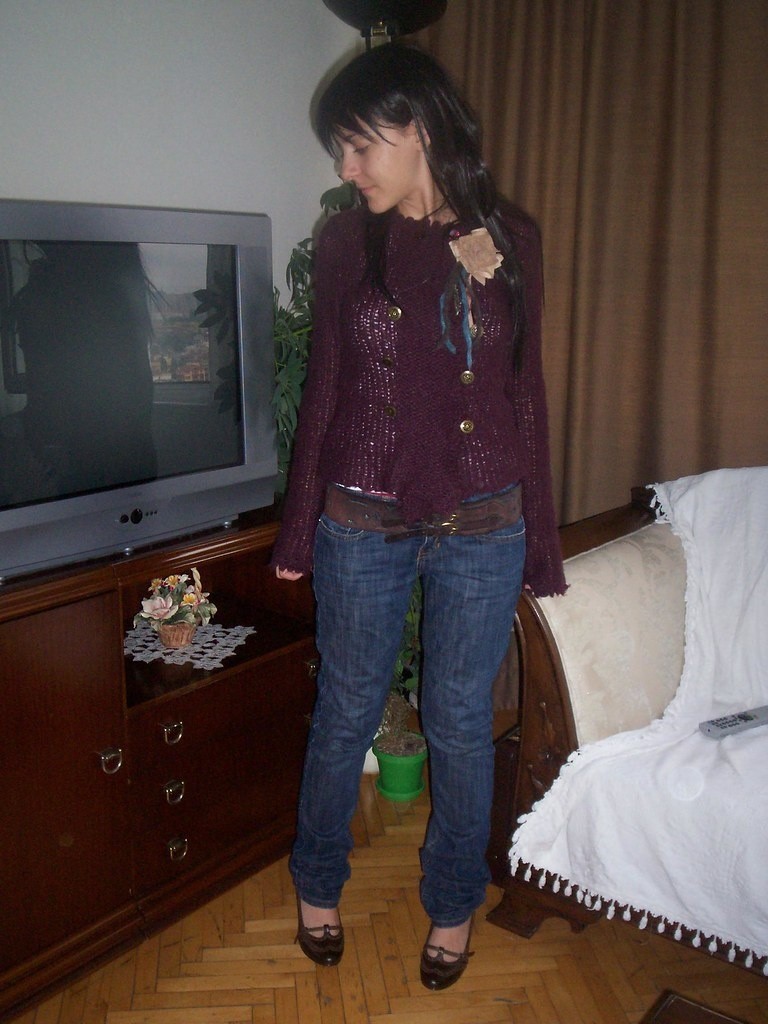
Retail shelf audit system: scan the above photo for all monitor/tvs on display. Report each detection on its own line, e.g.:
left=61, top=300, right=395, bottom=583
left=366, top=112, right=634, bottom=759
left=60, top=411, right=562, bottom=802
left=0, top=198, right=279, bottom=580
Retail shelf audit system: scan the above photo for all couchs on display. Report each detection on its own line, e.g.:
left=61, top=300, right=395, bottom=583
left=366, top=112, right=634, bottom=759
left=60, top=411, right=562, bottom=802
left=485, top=467, right=768, bottom=980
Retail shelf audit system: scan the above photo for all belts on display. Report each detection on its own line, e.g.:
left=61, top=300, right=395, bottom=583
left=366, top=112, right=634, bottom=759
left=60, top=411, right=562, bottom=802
left=320, top=480, right=523, bottom=544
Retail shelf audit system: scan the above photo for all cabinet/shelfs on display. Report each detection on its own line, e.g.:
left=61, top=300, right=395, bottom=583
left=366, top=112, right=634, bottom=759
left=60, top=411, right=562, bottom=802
left=0, top=492, right=319, bottom=1024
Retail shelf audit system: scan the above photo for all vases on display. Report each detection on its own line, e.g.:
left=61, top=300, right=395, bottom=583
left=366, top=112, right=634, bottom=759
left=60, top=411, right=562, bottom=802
left=155, top=617, right=202, bottom=648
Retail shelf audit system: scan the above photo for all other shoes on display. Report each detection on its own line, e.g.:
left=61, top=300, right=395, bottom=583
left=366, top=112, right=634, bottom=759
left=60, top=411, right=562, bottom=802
left=420, top=910, right=476, bottom=991
left=294, top=891, right=345, bottom=966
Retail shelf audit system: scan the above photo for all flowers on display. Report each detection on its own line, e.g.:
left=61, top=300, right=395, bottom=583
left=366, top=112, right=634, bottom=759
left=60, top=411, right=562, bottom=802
left=131, top=568, right=216, bottom=636
left=447, top=227, right=504, bottom=286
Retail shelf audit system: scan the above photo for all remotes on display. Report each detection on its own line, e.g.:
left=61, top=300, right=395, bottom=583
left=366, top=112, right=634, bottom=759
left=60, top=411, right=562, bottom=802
left=699, top=705, right=768, bottom=739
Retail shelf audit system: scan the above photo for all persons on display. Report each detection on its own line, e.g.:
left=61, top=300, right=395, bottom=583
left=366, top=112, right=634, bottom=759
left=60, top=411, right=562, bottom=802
left=14, top=240, right=159, bottom=495
left=272, top=45, right=566, bottom=990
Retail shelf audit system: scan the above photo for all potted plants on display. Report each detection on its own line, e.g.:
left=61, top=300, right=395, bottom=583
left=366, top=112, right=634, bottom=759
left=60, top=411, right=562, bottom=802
left=272, top=178, right=428, bottom=802
